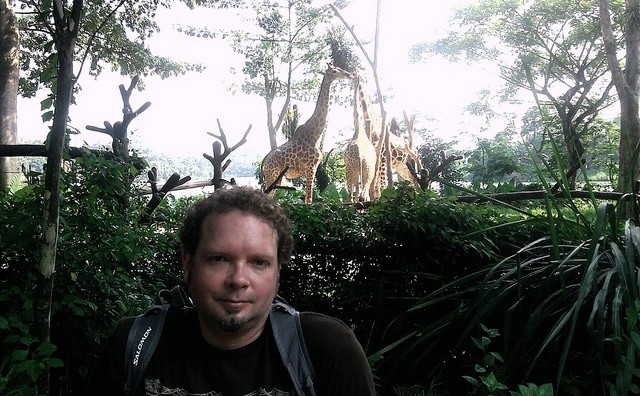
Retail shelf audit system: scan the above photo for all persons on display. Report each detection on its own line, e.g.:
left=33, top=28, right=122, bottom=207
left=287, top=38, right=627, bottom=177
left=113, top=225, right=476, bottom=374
left=81, top=183, right=378, bottom=396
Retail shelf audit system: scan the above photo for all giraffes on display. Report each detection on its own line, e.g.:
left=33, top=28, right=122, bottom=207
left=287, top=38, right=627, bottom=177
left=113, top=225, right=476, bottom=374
left=357, top=81, right=418, bottom=199
left=259, top=61, right=354, bottom=206
left=345, top=72, right=377, bottom=204
